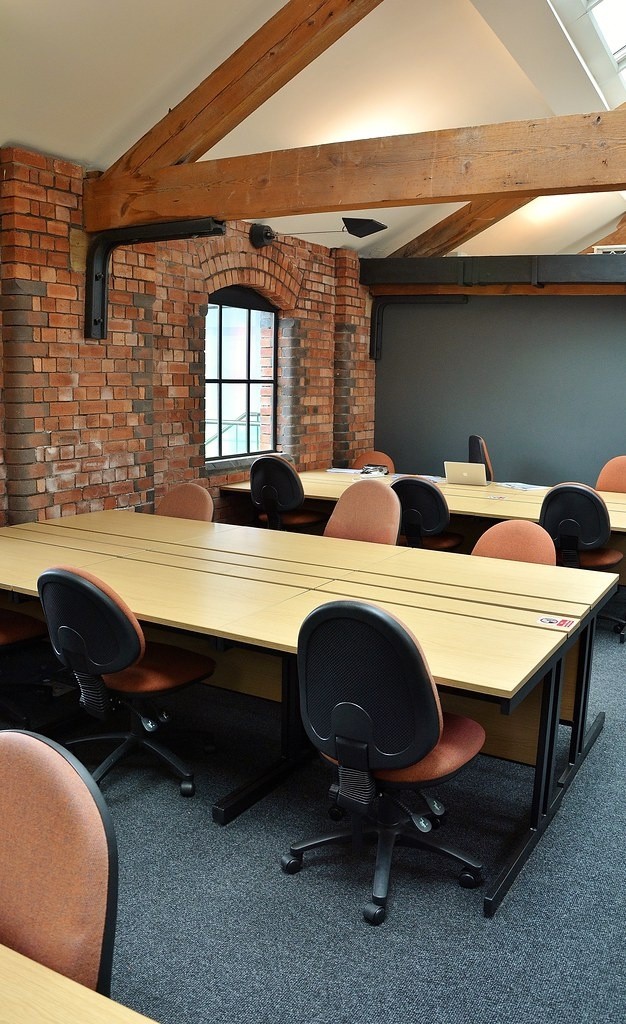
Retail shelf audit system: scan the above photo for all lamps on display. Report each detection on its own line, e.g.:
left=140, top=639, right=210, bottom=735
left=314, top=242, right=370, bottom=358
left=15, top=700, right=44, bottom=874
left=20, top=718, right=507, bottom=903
left=249, top=217, right=388, bottom=249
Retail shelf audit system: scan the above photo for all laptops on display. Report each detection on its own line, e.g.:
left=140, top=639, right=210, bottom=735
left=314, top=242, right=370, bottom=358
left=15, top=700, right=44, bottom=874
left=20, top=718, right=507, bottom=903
left=444, top=461, right=492, bottom=486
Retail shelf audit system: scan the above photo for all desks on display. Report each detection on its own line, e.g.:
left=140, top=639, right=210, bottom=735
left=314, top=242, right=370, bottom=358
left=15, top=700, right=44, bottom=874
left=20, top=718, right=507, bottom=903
left=220, top=468, right=626, bottom=533
left=0, top=511, right=619, bottom=919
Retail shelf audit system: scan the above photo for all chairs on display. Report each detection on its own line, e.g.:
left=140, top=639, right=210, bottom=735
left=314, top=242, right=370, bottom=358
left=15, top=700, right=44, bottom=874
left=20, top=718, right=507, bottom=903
left=539, top=482, right=626, bottom=634
left=38, top=568, right=217, bottom=798
left=469, top=435, right=493, bottom=481
left=470, top=520, right=559, bottom=566
left=250, top=456, right=331, bottom=532
left=323, top=480, right=401, bottom=546
left=0, top=728, right=118, bottom=997
left=391, top=475, right=464, bottom=553
left=352, top=451, right=395, bottom=473
left=595, top=455, right=626, bottom=493
left=281, top=602, right=485, bottom=925
left=156, top=484, right=214, bottom=523
left=0, top=610, right=52, bottom=726
